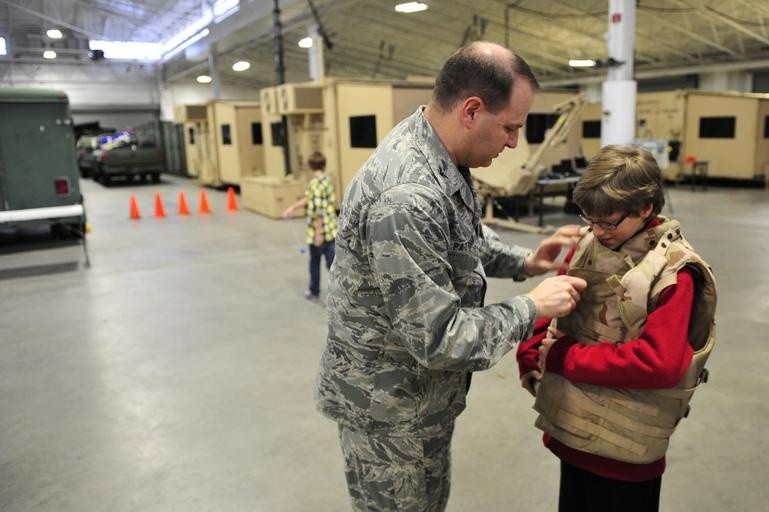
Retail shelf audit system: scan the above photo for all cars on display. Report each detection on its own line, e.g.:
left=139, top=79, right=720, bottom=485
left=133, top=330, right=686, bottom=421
left=69, top=129, right=167, bottom=188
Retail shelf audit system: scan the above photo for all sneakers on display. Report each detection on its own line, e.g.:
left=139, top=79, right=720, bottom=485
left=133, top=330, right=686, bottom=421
left=305, top=291, right=318, bottom=301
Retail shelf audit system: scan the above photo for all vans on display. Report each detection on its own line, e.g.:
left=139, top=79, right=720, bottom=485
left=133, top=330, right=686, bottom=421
left=1, top=87, right=87, bottom=242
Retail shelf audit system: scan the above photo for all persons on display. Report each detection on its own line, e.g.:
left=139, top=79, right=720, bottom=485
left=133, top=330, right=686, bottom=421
left=283, top=151, right=340, bottom=300
left=313, top=42, right=588, bottom=512
left=515, top=145, right=717, bottom=511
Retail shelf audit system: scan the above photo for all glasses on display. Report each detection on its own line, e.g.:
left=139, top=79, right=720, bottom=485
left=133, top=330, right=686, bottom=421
left=579, top=208, right=632, bottom=231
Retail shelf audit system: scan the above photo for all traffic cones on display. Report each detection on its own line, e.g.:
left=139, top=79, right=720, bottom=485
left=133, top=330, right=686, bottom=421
left=176, top=192, right=192, bottom=215
left=126, top=192, right=143, bottom=222
left=224, top=183, right=242, bottom=213
left=195, top=189, right=211, bottom=214
left=150, top=192, right=166, bottom=218
